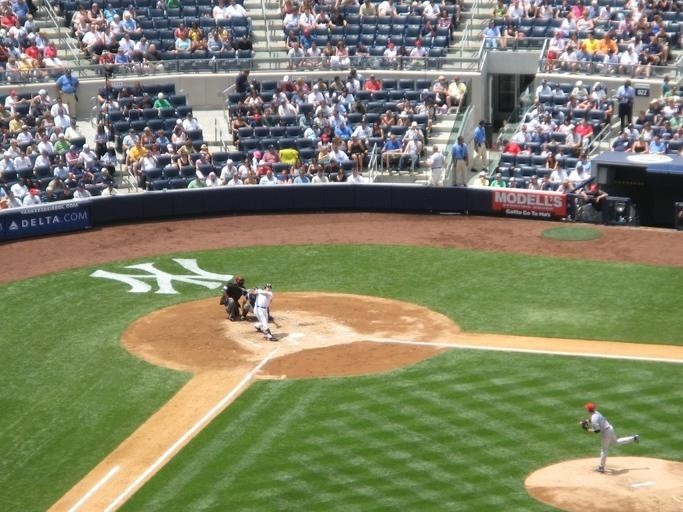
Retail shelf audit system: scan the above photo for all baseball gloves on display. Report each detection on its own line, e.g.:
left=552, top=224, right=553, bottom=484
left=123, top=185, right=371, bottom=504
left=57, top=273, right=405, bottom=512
left=581, top=420, right=588, bottom=428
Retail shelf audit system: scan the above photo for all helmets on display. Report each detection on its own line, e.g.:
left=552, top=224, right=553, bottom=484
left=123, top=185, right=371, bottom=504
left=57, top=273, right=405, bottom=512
left=264, top=283, right=273, bottom=289
left=236, top=276, right=244, bottom=287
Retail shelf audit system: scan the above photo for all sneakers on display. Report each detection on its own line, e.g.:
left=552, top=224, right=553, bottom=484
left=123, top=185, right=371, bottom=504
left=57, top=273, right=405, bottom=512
left=264, top=333, right=277, bottom=341
left=595, top=467, right=604, bottom=473
left=634, top=435, right=639, bottom=443
left=254, top=324, right=261, bottom=331
left=228, top=315, right=246, bottom=322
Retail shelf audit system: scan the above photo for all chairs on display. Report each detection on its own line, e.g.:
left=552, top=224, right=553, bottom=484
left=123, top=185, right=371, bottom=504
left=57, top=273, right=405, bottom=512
left=0, top=0, right=682, bottom=210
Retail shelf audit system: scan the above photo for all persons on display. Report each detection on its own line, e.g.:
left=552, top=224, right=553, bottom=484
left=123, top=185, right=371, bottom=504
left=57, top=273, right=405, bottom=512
left=242, top=68, right=467, bottom=185
left=491, top=79, right=683, bottom=231
left=484, top=1, right=683, bottom=79
left=578, top=402, right=642, bottom=475
left=470, top=120, right=489, bottom=172
left=278, top=1, right=467, bottom=71
left=474, top=172, right=488, bottom=186
left=1, top=2, right=252, bottom=209
left=218, top=272, right=249, bottom=323
left=246, top=281, right=280, bottom=344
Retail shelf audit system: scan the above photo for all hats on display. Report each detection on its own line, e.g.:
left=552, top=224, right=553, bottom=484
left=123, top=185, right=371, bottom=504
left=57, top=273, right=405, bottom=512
left=585, top=403, right=595, bottom=411
